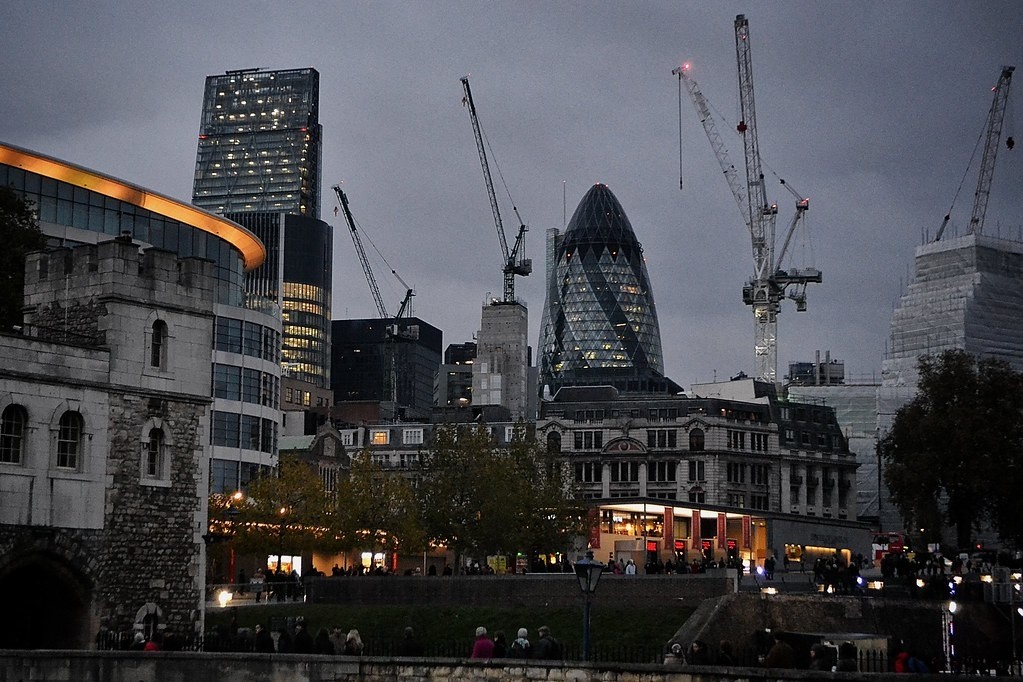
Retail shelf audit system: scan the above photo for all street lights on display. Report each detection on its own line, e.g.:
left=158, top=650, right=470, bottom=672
left=573, top=550, right=606, bottom=658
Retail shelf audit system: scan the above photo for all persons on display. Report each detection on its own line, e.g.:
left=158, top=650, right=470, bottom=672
left=332, top=564, right=398, bottom=576
left=130, top=627, right=182, bottom=650
left=812, top=552, right=868, bottom=582
left=800, top=555, right=806, bottom=574
left=644, top=557, right=745, bottom=575
left=470, top=626, right=559, bottom=660
left=254, top=616, right=364, bottom=656
left=399, top=627, right=420, bottom=656
left=607, top=558, right=638, bottom=575
left=764, top=555, right=775, bottom=580
left=896, top=646, right=927, bottom=673
left=835, top=643, right=857, bottom=672
left=881, top=551, right=976, bottom=578
left=428, top=562, right=453, bottom=576
left=237, top=566, right=300, bottom=603
left=460, top=563, right=494, bottom=575
left=535, top=559, right=574, bottom=572
left=765, top=632, right=793, bottom=667
left=783, top=554, right=790, bottom=570
left=661, top=639, right=736, bottom=666
left=809, top=643, right=823, bottom=669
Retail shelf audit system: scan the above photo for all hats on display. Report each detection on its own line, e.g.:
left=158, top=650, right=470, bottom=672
left=130, top=624, right=141, bottom=630
left=517, top=628, right=528, bottom=638
left=297, top=621, right=307, bottom=627
left=475, top=626, right=487, bottom=637
left=215, top=624, right=226, bottom=633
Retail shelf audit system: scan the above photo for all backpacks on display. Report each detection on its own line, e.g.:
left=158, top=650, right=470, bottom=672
left=512, top=642, right=524, bottom=659
left=544, top=637, right=562, bottom=660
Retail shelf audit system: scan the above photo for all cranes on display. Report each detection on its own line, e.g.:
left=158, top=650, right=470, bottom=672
left=331, top=183, right=417, bottom=322
left=670, top=13, right=823, bottom=396
left=931, top=63, right=1018, bottom=236
left=458, top=74, right=535, bottom=304
